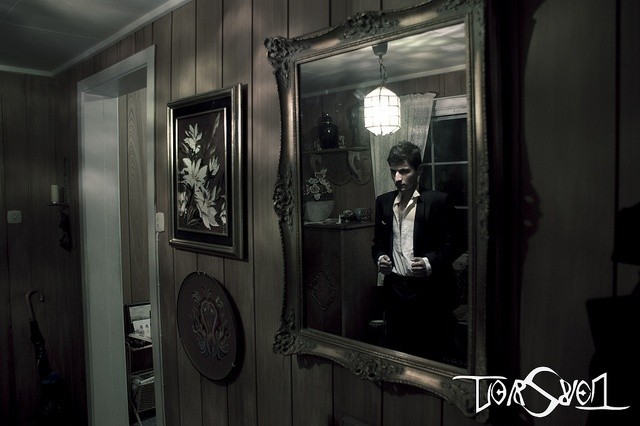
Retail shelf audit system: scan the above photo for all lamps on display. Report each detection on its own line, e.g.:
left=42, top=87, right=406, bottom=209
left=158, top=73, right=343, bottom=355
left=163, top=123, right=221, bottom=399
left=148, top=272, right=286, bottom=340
left=363, top=43, right=406, bottom=137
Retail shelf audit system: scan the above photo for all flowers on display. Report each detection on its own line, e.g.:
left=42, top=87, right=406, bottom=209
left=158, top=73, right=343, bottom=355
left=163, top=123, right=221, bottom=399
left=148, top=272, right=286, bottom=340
left=302, top=166, right=333, bottom=200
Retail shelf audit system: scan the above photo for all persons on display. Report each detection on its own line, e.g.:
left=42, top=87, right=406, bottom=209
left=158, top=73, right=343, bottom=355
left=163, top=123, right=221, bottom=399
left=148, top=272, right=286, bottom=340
left=371, top=140, right=458, bottom=353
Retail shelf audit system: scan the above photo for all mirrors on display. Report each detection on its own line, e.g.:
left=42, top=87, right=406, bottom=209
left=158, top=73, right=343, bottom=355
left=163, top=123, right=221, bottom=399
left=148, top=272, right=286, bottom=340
left=262, top=0, right=502, bottom=425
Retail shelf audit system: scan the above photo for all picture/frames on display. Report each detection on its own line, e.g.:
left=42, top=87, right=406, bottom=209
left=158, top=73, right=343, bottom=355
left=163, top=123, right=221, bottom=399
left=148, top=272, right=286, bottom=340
left=167, top=83, right=247, bottom=262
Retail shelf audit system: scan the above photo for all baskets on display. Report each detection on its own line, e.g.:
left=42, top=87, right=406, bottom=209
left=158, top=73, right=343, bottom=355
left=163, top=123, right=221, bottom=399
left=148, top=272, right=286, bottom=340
left=133, top=370, right=155, bottom=410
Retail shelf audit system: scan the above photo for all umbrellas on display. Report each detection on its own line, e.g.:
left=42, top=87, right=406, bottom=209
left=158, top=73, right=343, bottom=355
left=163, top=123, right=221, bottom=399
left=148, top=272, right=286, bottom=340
left=25, top=289, right=51, bottom=387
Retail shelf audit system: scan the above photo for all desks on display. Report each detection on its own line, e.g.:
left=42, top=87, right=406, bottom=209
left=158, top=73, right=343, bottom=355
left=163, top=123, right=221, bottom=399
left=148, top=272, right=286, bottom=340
left=303, top=218, right=376, bottom=340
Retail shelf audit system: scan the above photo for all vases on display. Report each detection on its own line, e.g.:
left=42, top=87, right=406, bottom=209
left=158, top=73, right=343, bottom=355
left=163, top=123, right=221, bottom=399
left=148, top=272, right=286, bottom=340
left=303, top=200, right=334, bottom=221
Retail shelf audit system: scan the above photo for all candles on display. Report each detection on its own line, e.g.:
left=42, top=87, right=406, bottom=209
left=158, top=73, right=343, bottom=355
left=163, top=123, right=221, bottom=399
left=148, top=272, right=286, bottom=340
left=50, top=183, right=59, bottom=202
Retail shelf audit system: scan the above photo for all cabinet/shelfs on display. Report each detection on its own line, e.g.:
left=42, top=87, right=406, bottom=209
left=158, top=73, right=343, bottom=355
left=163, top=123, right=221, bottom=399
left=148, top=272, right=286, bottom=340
left=303, top=146, right=373, bottom=186
left=127, top=336, right=157, bottom=425
